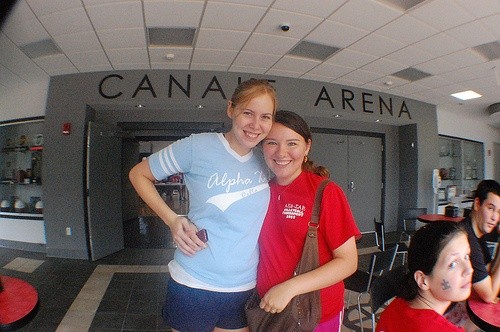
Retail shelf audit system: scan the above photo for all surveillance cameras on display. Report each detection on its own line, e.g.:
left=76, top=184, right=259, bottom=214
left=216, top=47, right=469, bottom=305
left=280, top=23, right=290, bottom=31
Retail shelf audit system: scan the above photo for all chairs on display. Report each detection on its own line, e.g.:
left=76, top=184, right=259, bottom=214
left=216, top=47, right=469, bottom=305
left=343, top=208, right=427, bottom=332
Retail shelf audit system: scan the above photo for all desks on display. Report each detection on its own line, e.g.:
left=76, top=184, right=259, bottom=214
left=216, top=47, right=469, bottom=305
left=417, top=214, right=465, bottom=222
left=0, top=275, right=40, bottom=332
left=466, top=289, right=500, bottom=332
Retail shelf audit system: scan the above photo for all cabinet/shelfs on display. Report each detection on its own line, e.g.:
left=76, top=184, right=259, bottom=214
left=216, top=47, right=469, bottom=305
left=0, top=115, right=45, bottom=221
left=438, top=133, right=486, bottom=206
left=156, top=182, right=188, bottom=200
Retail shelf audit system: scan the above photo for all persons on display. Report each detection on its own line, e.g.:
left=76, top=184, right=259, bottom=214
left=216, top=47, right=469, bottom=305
left=129, top=78, right=277, bottom=332
left=376, top=180, right=500, bottom=332
left=256, top=111, right=361, bottom=332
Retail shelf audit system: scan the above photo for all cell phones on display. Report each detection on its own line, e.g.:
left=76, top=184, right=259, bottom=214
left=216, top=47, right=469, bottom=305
left=196, top=229, right=208, bottom=243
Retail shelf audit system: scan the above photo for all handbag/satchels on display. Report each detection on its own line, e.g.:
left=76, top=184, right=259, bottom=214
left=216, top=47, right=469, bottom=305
left=244, top=226, right=322, bottom=331
